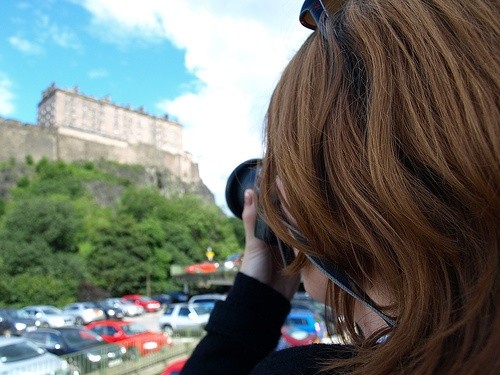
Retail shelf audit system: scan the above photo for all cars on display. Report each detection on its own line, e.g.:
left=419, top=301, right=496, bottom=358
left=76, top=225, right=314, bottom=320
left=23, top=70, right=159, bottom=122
left=0, top=336, right=81, bottom=375
left=0, top=287, right=360, bottom=375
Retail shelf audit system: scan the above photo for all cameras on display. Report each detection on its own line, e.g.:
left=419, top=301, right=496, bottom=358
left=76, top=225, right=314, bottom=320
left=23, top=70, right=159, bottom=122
left=224, top=159, right=286, bottom=247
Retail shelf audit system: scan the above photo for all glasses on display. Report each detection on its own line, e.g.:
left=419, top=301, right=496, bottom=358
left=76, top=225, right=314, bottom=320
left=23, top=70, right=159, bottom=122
left=299, top=0, right=357, bottom=75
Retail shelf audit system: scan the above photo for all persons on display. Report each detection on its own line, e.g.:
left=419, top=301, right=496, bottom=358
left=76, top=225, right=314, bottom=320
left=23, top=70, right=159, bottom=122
left=179, top=0, right=500, bottom=375
